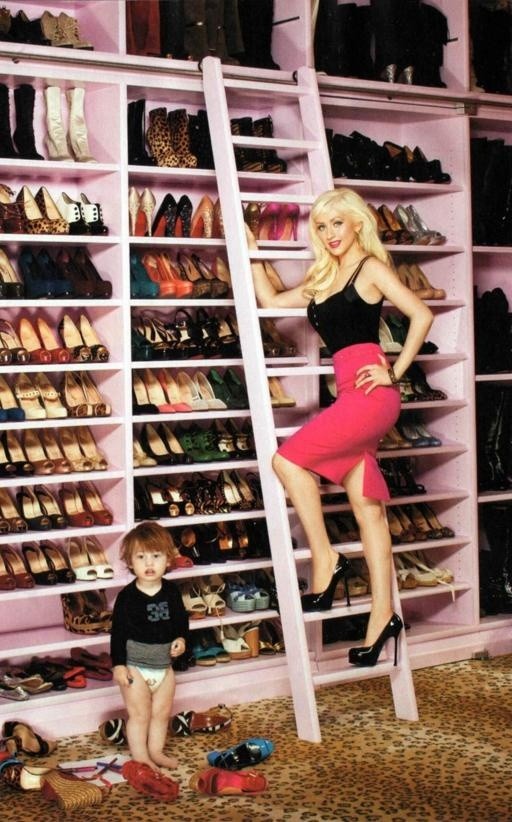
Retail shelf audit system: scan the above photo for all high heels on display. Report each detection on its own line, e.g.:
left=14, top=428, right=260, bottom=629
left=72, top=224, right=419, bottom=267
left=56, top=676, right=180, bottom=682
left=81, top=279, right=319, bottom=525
left=266, top=321, right=297, bottom=355
left=0, top=427, right=109, bottom=478
left=0, top=183, right=109, bottom=235
left=0, top=6, right=94, bottom=51
left=189, top=620, right=286, bottom=667
left=381, top=414, right=443, bottom=448
left=129, top=186, right=225, bottom=238
left=325, top=514, right=362, bottom=543
left=0, top=735, right=22, bottom=757
left=1, top=720, right=58, bottom=757
left=349, top=613, right=403, bottom=667
left=132, top=469, right=264, bottom=522
left=323, top=128, right=451, bottom=185
left=260, top=326, right=281, bottom=357
left=132, top=418, right=257, bottom=467
left=132, top=307, right=242, bottom=361
left=241, top=201, right=300, bottom=242
left=0, top=535, right=116, bottom=591
left=130, top=243, right=232, bottom=299
left=377, top=458, right=427, bottom=496
left=132, top=367, right=250, bottom=413
left=120, top=760, right=178, bottom=802
left=395, top=261, right=446, bottom=300
left=393, top=363, right=447, bottom=401
left=366, top=203, right=447, bottom=247
left=207, top=737, right=274, bottom=770
left=0, top=314, right=109, bottom=365
left=0, top=245, right=112, bottom=300
left=188, top=767, right=269, bottom=796
left=381, top=505, right=455, bottom=544
left=0, top=482, right=119, bottom=535
left=268, top=378, right=299, bottom=407
left=0, top=371, right=111, bottom=421
left=378, top=313, right=438, bottom=354
left=301, top=554, right=353, bottom=611
left=173, top=523, right=271, bottom=568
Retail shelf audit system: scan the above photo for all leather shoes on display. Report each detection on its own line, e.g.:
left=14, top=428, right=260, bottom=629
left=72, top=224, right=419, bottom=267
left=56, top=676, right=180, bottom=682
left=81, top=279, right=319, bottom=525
left=0, top=647, right=113, bottom=700
left=1, top=762, right=46, bottom=790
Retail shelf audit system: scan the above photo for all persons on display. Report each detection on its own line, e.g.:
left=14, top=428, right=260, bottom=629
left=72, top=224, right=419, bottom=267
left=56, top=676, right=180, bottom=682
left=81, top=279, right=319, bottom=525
left=112, top=522, right=187, bottom=772
left=243, top=188, right=434, bottom=665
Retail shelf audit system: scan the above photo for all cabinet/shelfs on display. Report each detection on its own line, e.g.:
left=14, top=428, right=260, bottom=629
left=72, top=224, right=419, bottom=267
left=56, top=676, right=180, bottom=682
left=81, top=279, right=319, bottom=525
left=1, top=3, right=511, bottom=98
left=473, top=101, right=511, bottom=661
left=1, top=57, right=472, bottom=748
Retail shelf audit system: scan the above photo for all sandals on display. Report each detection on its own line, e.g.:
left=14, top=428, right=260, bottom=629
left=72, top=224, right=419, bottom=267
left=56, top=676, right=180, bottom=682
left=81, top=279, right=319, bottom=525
left=332, top=558, right=371, bottom=599
left=169, top=704, right=234, bottom=736
left=41, top=756, right=118, bottom=811
left=180, top=570, right=278, bottom=621
left=96, top=719, right=127, bottom=747
left=60, top=594, right=104, bottom=634
left=392, top=550, right=455, bottom=588
left=82, top=590, right=113, bottom=632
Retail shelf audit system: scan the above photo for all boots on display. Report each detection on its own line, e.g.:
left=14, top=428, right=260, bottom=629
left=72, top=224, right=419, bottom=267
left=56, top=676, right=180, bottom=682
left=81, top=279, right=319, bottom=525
left=65, top=88, right=98, bottom=163
left=42, top=86, right=75, bottom=162
left=468, top=0, right=511, bottom=614
left=318, top=1, right=450, bottom=85
left=126, top=0, right=279, bottom=68
left=13, top=85, right=44, bottom=159
left=0, top=83, right=16, bottom=158
left=127, top=98, right=287, bottom=173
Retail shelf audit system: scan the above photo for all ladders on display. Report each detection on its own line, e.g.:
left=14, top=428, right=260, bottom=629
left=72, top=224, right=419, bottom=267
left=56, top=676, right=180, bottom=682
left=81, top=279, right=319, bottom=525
left=201, top=56, right=419, bottom=745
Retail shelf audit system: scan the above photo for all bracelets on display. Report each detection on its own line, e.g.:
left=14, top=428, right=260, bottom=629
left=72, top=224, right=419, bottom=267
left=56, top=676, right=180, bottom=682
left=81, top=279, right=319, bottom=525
left=388, top=367, right=400, bottom=387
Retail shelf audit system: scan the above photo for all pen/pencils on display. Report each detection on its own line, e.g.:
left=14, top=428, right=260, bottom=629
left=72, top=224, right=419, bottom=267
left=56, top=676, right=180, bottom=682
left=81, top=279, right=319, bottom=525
left=95, top=757, right=122, bottom=778
left=60, top=767, right=98, bottom=772
